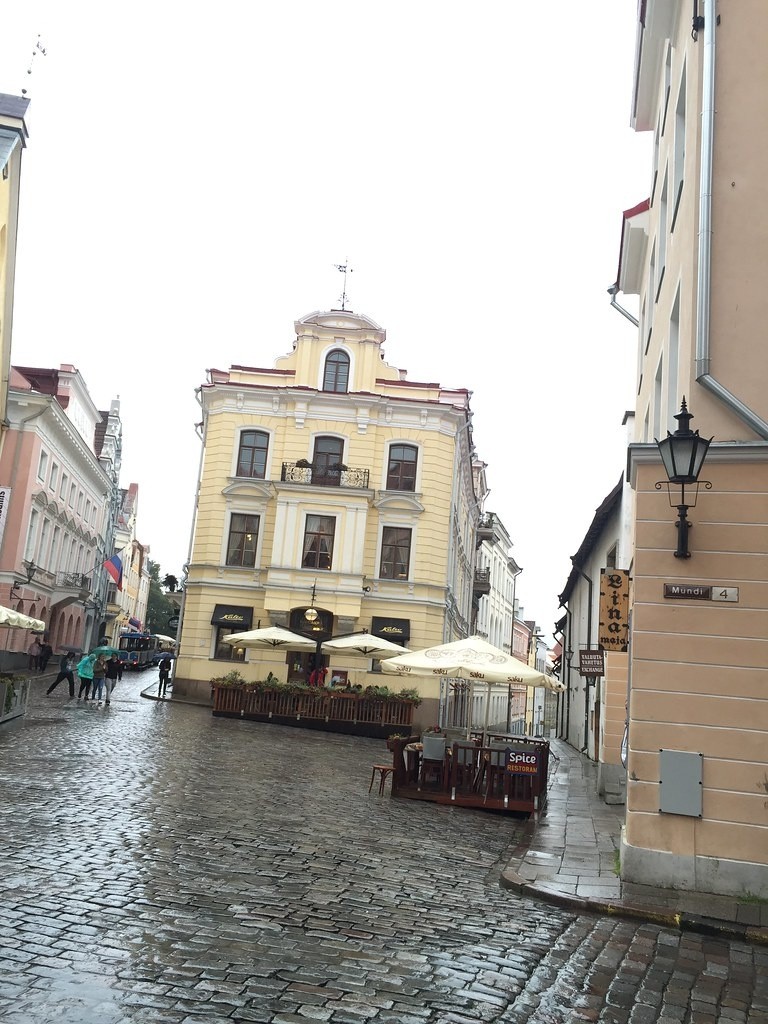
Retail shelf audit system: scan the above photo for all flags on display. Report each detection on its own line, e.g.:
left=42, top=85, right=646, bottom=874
left=102, top=550, right=123, bottom=592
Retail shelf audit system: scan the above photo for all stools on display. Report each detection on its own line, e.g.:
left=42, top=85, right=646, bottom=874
left=368, top=764, right=397, bottom=798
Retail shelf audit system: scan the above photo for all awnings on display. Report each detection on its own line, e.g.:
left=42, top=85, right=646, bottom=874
left=0, top=605, right=45, bottom=632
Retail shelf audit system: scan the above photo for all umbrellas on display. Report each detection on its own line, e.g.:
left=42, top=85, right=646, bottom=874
left=223, top=627, right=317, bottom=678
left=379, top=635, right=567, bottom=747
left=105, top=635, right=113, bottom=640
left=57, top=644, right=83, bottom=658
left=92, top=646, right=121, bottom=664
left=30, top=629, right=51, bottom=641
left=152, top=652, right=177, bottom=668
left=320, top=634, right=414, bottom=689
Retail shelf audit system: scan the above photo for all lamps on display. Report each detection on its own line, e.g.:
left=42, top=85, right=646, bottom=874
left=585, top=675, right=596, bottom=686
left=653, top=395, right=714, bottom=557
left=565, top=645, right=574, bottom=660
left=92, top=593, right=101, bottom=604
left=26, top=559, right=36, bottom=582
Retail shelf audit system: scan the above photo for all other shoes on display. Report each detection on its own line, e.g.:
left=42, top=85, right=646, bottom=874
left=46, top=694, right=50, bottom=697
left=99, top=702, right=102, bottom=706
left=78, top=697, right=81, bottom=699
left=84, top=698, right=89, bottom=701
left=107, top=702, right=110, bottom=705
left=158, top=693, right=161, bottom=696
left=70, top=696, right=76, bottom=699
left=163, top=693, right=166, bottom=697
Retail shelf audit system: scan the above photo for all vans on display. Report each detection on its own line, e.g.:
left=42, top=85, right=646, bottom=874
left=116, top=631, right=159, bottom=669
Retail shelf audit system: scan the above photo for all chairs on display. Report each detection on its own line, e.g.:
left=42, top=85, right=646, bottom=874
left=417, top=730, right=475, bottom=793
left=484, top=739, right=536, bottom=798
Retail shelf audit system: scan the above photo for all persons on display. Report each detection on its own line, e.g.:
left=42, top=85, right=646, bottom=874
left=92, top=653, right=108, bottom=703
left=76, top=654, right=97, bottom=700
left=106, top=653, right=122, bottom=705
left=97, top=638, right=108, bottom=647
left=38, top=639, right=52, bottom=673
left=318, top=670, right=324, bottom=687
left=309, top=668, right=317, bottom=685
left=158, top=659, right=171, bottom=696
left=47, top=652, right=77, bottom=697
left=28, top=638, right=41, bottom=670
left=332, top=681, right=340, bottom=690
left=346, top=679, right=380, bottom=692
